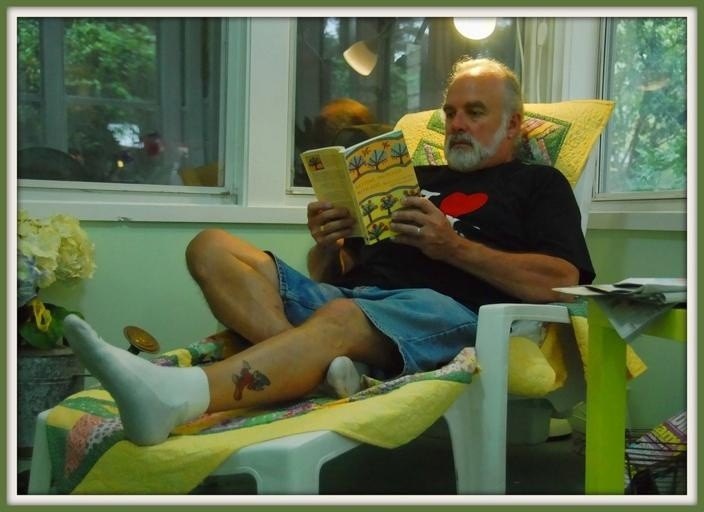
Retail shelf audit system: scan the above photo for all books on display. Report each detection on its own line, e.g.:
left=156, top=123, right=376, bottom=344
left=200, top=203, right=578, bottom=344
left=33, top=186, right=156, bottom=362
left=299, top=127, right=429, bottom=248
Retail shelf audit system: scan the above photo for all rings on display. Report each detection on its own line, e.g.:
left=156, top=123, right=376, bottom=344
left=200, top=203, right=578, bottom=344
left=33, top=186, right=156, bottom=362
left=415, top=227, right=421, bottom=238
left=319, top=226, right=325, bottom=235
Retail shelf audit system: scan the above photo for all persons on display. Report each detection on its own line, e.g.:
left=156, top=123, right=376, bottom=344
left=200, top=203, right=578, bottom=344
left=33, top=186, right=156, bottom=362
left=62, top=56, right=599, bottom=453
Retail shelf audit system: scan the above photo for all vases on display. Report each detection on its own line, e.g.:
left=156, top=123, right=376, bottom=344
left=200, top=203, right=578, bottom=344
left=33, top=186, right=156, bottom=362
left=18, top=346, right=92, bottom=459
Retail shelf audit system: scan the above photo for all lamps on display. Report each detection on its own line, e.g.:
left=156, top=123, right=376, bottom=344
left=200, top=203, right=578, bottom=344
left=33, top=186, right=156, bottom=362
left=341, top=18, right=405, bottom=78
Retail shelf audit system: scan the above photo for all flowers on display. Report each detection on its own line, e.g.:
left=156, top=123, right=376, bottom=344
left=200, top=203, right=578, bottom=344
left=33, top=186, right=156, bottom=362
left=17, top=212, right=101, bottom=349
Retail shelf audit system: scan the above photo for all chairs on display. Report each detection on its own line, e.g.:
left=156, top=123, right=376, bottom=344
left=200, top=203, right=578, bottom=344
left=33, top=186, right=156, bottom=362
left=28, top=139, right=601, bottom=497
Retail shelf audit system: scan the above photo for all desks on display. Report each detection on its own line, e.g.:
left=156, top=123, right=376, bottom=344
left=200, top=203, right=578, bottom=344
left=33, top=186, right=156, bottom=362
left=586, top=299, right=685, bottom=503
